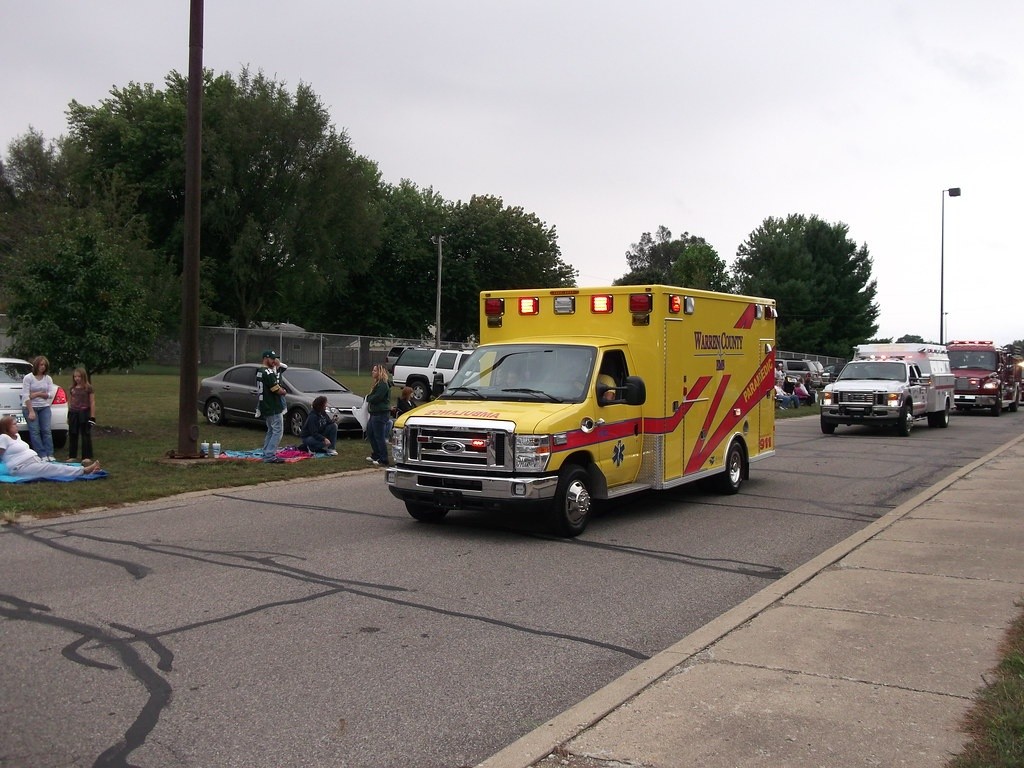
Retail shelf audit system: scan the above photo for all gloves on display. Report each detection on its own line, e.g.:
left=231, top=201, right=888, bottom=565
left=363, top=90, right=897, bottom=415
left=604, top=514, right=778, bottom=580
left=87, top=416, right=96, bottom=427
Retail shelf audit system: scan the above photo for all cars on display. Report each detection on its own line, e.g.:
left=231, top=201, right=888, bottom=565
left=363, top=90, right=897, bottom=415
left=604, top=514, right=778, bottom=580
left=0, top=357, right=69, bottom=450
left=196, top=364, right=372, bottom=436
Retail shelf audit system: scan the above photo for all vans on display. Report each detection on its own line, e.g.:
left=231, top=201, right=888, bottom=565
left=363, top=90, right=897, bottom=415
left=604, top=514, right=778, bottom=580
left=385, top=346, right=422, bottom=375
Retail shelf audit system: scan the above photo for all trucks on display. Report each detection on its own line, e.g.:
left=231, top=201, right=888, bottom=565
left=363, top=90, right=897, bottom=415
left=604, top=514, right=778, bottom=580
left=819, top=343, right=955, bottom=436
left=385, top=285, right=777, bottom=537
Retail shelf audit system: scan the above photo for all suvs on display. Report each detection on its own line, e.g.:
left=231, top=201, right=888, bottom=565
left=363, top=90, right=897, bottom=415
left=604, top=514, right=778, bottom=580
left=777, top=358, right=844, bottom=387
left=392, top=346, right=474, bottom=401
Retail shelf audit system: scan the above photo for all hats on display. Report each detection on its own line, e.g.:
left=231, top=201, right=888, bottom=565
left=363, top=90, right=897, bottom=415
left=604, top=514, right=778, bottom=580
left=262, top=350, right=280, bottom=360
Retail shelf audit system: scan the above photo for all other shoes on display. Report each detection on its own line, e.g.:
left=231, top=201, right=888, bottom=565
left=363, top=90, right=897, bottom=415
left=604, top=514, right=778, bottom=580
left=42, top=456, right=56, bottom=462
left=366, top=457, right=384, bottom=465
left=306, top=445, right=315, bottom=454
left=324, top=449, right=337, bottom=455
left=271, top=458, right=284, bottom=463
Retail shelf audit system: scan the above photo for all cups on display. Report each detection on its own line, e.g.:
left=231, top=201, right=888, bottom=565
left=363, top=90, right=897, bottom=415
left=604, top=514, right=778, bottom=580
left=201, top=443, right=209, bottom=457
left=213, top=443, right=221, bottom=458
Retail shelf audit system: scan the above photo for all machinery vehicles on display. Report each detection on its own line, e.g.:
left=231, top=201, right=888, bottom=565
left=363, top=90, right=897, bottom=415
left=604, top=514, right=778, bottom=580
left=948, top=340, right=1024, bottom=416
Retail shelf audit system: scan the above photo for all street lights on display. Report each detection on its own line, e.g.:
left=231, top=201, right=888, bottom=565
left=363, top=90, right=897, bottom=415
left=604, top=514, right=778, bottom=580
left=940, top=187, right=961, bottom=343
left=431, top=235, right=451, bottom=345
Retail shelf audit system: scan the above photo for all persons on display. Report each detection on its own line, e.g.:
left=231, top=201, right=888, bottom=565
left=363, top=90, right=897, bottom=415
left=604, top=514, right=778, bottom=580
left=257, top=350, right=286, bottom=464
left=397, top=387, right=418, bottom=415
left=301, top=396, right=339, bottom=457
left=772, top=379, right=801, bottom=408
left=255, top=355, right=288, bottom=419
left=385, top=406, right=399, bottom=443
left=0, top=415, right=102, bottom=480
left=797, top=378, right=813, bottom=405
left=805, top=371, right=816, bottom=403
left=775, top=363, right=785, bottom=386
left=555, top=355, right=618, bottom=401
left=67, top=366, right=96, bottom=466
left=22, top=355, right=56, bottom=463
left=775, top=389, right=793, bottom=411
left=364, top=363, right=391, bottom=465
left=493, top=366, right=523, bottom=392
left=794, top=381, right=810, bottom=406
